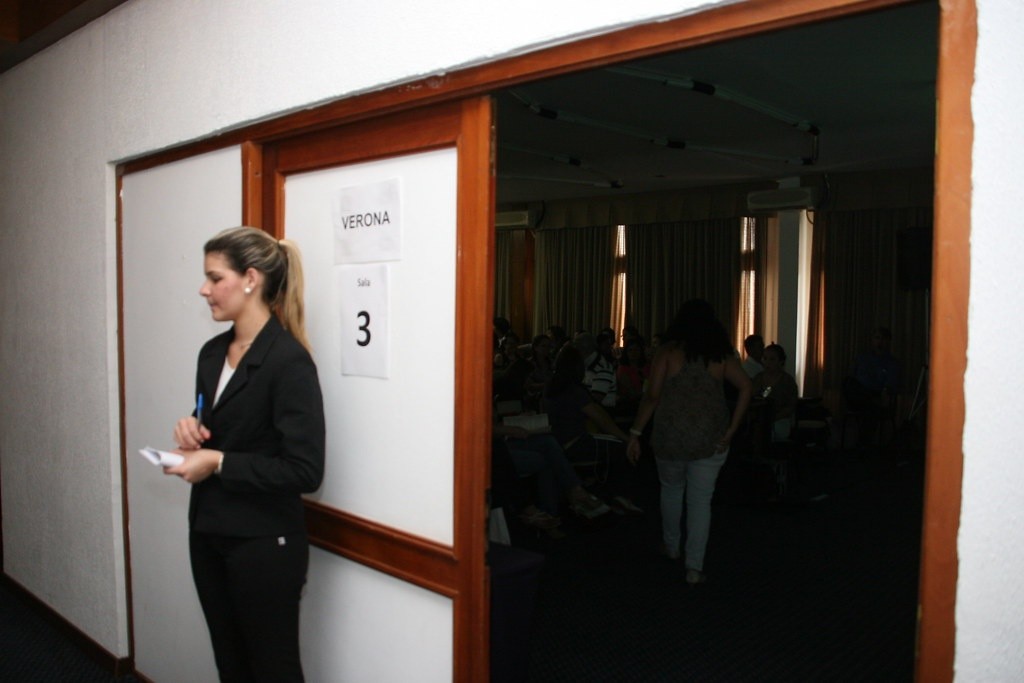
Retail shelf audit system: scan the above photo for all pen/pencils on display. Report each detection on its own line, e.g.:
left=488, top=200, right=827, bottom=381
left=195, top=392, right=204, bottom=432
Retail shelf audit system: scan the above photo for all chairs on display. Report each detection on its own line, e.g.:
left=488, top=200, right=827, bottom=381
left=497, top=388, right=901, bottom=541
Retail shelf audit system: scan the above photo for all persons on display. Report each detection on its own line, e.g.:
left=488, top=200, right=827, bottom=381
left=494, top=317, right=664, bottom=529
left=848, top=326, right=902, bottom=448
left=626, top=298, right=753, bottom=589
left=162, top=226, right=326, bottom=683
left=744, top=335, right=799, bottom=444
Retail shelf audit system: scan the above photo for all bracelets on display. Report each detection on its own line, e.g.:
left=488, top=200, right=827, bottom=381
left=629, top=428, right=642, bottom=436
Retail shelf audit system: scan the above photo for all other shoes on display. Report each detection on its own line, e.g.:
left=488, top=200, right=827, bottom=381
left=665, top=550, right=680, bottom=560
left=568, top=489, right=602, bottom=511
left=686, top=570, right=707, bottom=583
left=517, top=506, right=561, bottom=529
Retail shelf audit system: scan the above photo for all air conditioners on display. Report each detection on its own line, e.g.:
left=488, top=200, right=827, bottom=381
left=495, top=209, right=537, bottom=231
left=746, top=185, right=817, bottom=214
